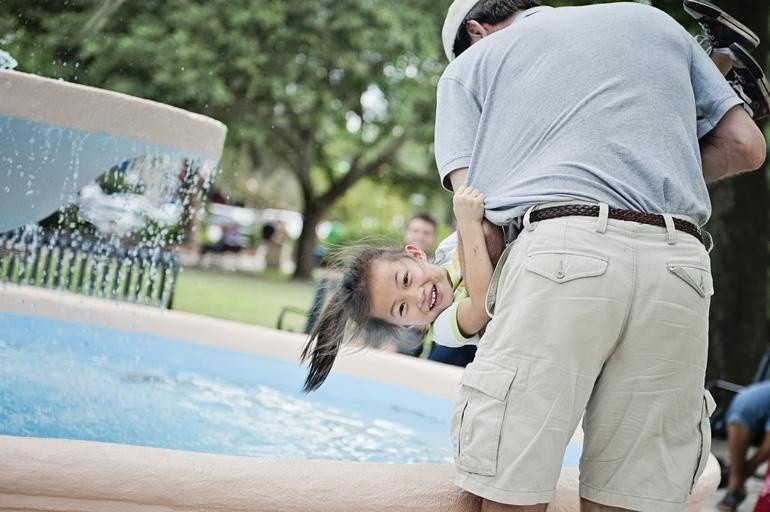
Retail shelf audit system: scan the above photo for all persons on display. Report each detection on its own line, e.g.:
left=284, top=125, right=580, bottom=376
left=417, top=0, right=770, bottom=512
left=383, top=213, right=438, bottom=360
left=296, top=182, right=509, bottom=396
left=717, top=378, right=770, bottom=512
left=427, top=226, right=482, bottom=369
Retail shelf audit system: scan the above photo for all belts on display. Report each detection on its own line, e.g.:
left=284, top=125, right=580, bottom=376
left=515, top=204, right=702, bottom=241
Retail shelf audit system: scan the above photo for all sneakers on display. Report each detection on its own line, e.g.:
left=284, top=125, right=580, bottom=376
left=683, top=0, right=760, bottom=54
left=725, top=42, right=770, bottom=122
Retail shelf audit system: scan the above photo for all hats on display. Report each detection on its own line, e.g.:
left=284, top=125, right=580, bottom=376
left=442, top=0, right=480, bottom=62
